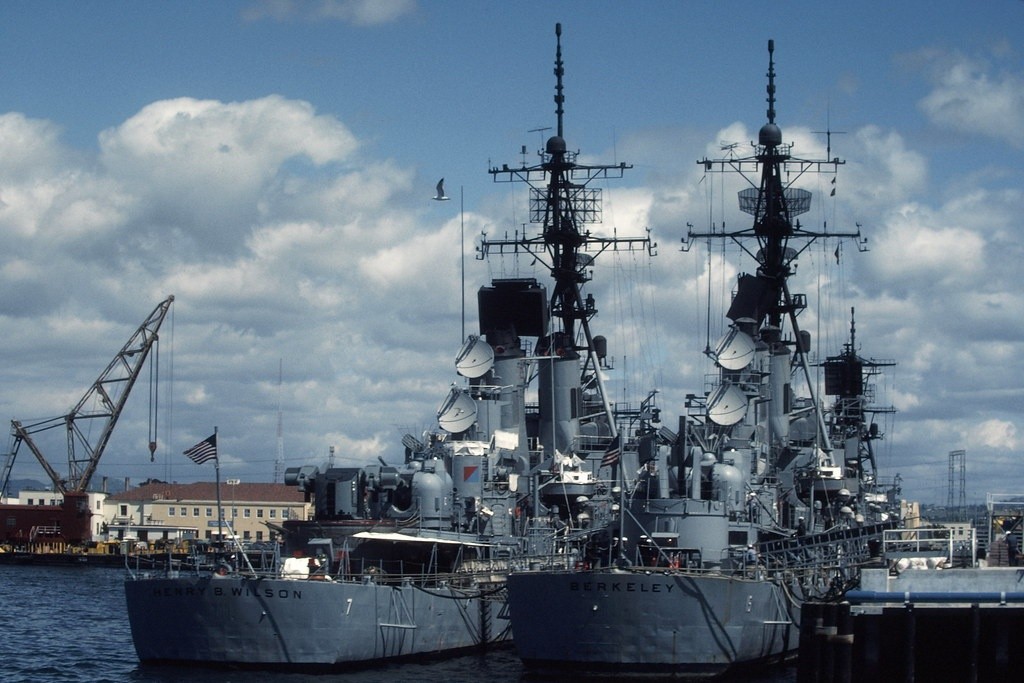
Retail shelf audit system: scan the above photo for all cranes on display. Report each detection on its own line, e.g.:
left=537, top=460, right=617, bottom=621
left=0, top=294, right=176, bottom=499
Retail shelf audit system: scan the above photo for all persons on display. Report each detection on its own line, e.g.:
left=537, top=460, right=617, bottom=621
left=1003, top=531, right=1017, bottom=567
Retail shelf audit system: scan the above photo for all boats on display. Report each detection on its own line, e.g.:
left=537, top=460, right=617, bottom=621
left=114, top=19, right=1022, bottom=683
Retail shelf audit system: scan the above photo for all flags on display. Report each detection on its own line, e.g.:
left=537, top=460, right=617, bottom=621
left=183, top=434, right=216, bottom=466
left=600, top=434, right=621, bottom=468
